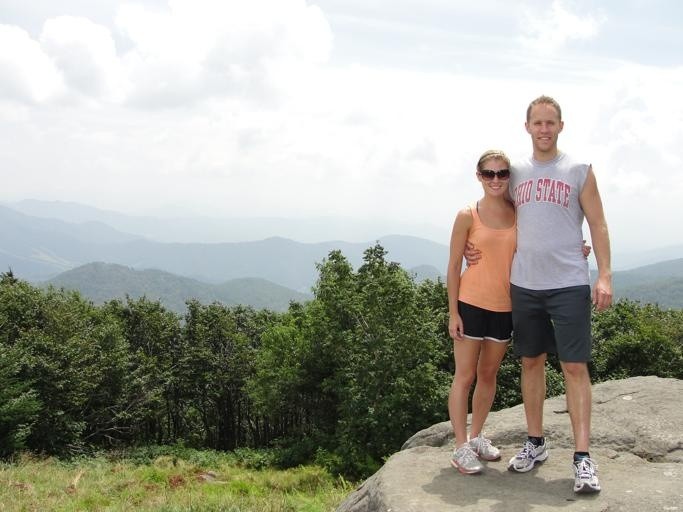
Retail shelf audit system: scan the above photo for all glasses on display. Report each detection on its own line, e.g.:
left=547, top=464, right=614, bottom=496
left=479, top=170, right=511, bottom=180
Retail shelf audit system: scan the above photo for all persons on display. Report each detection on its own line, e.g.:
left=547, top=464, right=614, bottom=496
left=503, top=95, right=614, bottom=495
left=446, top=149, right=591, bottom=475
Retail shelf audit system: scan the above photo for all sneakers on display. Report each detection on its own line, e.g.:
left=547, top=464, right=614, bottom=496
left=468, top=433, right=501, bottom=461
left=508, top=437, right=549, bottom=473
left=571, top=454, right=601, bottom=494
left=450, top=443, right=484, bottom=474
